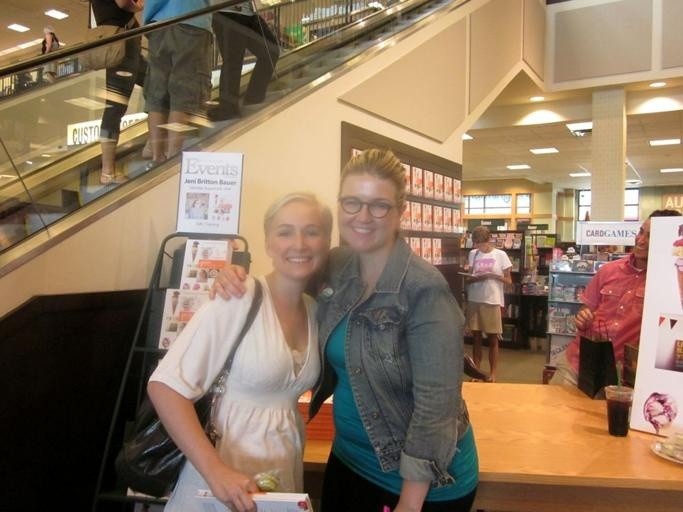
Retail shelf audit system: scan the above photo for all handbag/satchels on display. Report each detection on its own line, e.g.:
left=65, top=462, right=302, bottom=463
left=114, top=388, right=215, bottom=498
left=576, top=318, right=619, bottom=400
left=76, top=25, right=127, bottom=70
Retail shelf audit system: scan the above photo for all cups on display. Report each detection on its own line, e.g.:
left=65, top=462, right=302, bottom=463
left=604, top=386, right=635, bottom=437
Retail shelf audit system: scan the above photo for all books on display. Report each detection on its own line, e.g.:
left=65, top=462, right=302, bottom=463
left=481, top=227, right=555, bottom=341
left=158, top=288, right=215, bottom=349
left=396, top=161, right=466, bottom=269
left=457, top=231, right=473, bottom=337
left=250, top=490, right=314, bottom=512
left=179, top=237, right=233, bottom=292
left=456, top=270, right=493, bottom=279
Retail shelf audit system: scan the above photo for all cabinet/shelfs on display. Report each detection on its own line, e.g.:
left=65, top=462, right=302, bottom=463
left=461, top=211, right=606, bottom=384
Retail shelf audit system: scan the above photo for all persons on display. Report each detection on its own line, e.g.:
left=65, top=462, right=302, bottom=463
left=546, top=208, right=683, bottom=385
left=90, top=1, right=169, bottom=184
left=40, top=25, right=61, bottom=83
left=145, top=191, right=334, bottom=512
left=206, top=1, right=281, bottom=121
left=208, top=149, right=481, bottom=512
left=138, top=1, right=215, bottom=172
left=460, top=221, right=511, bottom=381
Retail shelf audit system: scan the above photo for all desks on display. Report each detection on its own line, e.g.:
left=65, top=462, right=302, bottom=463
left=296, top=381, right=683, bottom=512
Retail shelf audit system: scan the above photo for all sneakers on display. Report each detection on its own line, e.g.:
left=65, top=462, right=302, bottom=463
left=146, top=154, right=167, bottom=169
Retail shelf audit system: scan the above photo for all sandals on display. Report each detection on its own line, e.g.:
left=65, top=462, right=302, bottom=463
left=98, top=172, right=129, bottom=185
left=141, top=145, right=166, bottom=159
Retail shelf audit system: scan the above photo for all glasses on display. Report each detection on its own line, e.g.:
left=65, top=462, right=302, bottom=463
left=338, top=195, right=396, bottom=218
